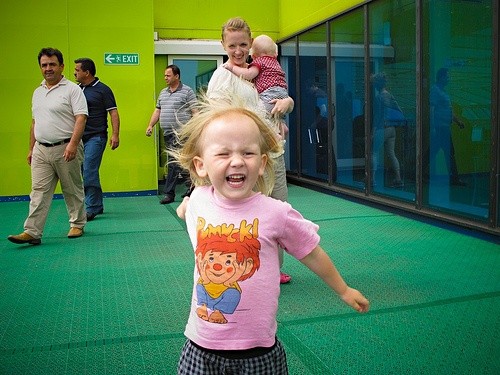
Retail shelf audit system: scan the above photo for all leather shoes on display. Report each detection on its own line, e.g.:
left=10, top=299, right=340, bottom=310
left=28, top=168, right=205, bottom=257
left=68, top=227, right=83, bottom=239
left=8, top=232, right=42, bottom=244
left=181, top=191, right=191, bottom=197
left=160, top=193, right=175, bottom=204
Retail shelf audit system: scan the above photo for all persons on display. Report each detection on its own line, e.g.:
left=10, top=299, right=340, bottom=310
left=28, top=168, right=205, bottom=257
left=73, top=57, right=120, bottom=222
left=222, top=34, right=289, bottom=142
left=146, top=65, right=199, bottom=204
left=205, top=17, right=294, bottom=283
left=304, top=81, right=338, bottom=182
left=427, top=67, right=467, bottom=187
left=372, top=71, right=406, bottom=187
left=161, top=85, right=369, bottom=375
left=8, top=47, right=88, bottom=245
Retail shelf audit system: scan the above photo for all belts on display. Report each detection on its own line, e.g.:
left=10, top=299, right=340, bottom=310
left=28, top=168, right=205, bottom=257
left=39, top=138, right=70, bottom=147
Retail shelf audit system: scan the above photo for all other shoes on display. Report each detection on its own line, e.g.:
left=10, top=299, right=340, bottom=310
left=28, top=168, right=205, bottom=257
left=86, top=208, right=104, bottom=222
left=280, top=270, right=291, bottom=283
